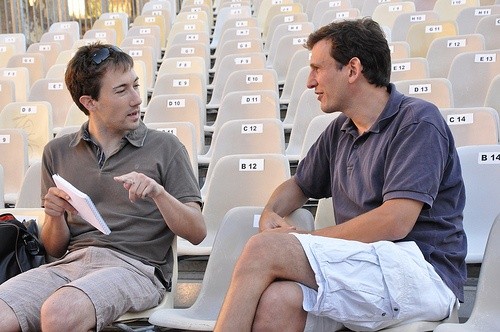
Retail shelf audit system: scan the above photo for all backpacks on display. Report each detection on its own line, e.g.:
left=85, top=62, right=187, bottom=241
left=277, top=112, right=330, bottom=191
left=0, top=213, right=46, bottom=285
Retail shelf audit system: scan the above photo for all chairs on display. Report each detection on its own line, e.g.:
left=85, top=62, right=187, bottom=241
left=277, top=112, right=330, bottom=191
left=0, top=0, right=500, bottom=332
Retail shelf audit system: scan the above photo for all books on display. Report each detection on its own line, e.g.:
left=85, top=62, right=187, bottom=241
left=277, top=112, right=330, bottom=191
left=52, top=173, right=111, bottom=235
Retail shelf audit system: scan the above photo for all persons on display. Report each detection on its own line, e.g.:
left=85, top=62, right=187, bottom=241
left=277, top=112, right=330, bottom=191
left=0, top=42, right=206, bottom=332
left=214, top=18, right=468, bottom=332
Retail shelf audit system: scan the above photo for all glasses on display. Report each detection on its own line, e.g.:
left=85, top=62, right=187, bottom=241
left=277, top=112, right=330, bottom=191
left=79, top=46, right=123, bottom=94
